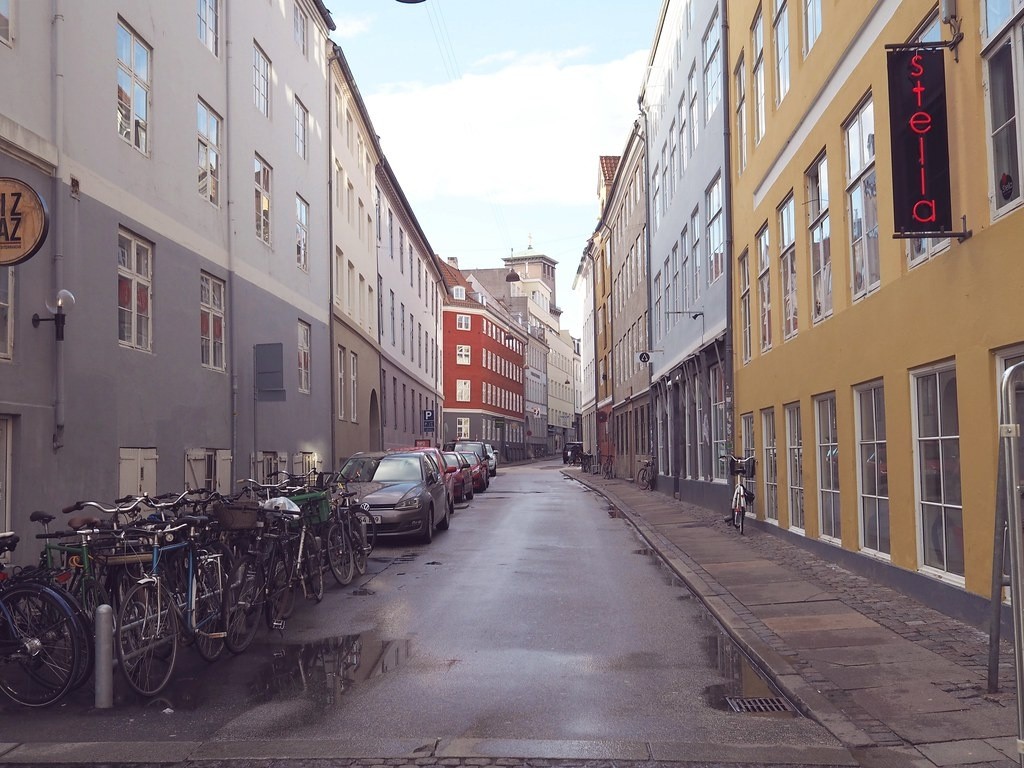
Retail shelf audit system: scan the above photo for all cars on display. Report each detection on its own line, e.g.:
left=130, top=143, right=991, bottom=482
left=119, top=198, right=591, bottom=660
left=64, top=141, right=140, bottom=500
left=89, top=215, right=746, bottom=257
left=332, top=451, right=449, bottom=544
left=486, top=444, right=498, bottom=477
left=442, top=452, right=473, bottom=502
left=386, top=447, right=457, bottom=514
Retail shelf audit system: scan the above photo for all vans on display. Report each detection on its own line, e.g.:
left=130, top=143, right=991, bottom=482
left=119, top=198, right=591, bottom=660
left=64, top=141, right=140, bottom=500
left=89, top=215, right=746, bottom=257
left=564, top=442, right=582, bottom=463
left=444, top=442, right=490, bottom=488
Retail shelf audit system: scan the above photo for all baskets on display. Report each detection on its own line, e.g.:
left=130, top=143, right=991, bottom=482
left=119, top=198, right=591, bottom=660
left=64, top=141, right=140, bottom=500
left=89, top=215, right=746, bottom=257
left=731, top=457, right=755, bottom=477
left=213, top=501, right=260, bottom=531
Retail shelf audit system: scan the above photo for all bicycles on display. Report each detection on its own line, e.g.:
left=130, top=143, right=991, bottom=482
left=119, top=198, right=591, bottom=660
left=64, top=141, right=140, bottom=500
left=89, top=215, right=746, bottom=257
left=0, top=462, right=379, bottom=708
left=601, top=454, right=615, bottom=479
left=719, top=454, right=758, bottom=536
left=637, top=457, right=657, bottom=491
